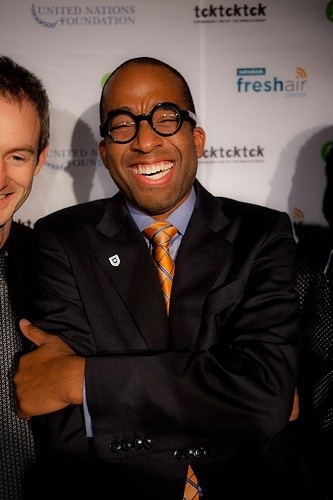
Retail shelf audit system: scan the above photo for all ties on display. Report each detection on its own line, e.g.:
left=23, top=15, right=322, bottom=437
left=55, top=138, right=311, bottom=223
left=143, top=221, right=202, bottom=500
left=0, top=256, right=35, bottom=500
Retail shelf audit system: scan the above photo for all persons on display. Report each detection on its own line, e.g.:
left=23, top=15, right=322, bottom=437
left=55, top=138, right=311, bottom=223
left=294, top=147, right=333, bottom=500
left=5, top=54, right=305, bottom=500
left=0, top=56, right=50, bottom=500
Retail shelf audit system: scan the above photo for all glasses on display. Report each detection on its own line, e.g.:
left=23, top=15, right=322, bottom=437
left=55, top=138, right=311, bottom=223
left=99, top=102, right=197, bottom=145
